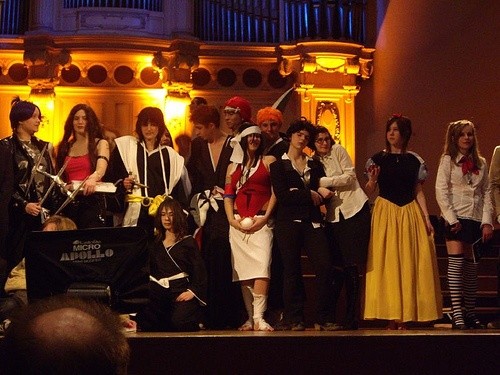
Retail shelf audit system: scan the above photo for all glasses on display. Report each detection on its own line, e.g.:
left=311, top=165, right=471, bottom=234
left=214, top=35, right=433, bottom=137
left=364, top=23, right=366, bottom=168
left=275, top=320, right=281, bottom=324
left=315, top=138, right=331, bottom=143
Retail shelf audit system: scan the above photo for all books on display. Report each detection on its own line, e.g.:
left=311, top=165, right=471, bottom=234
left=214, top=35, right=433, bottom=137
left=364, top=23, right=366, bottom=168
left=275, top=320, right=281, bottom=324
left=472, top=238, right=490, bottom=264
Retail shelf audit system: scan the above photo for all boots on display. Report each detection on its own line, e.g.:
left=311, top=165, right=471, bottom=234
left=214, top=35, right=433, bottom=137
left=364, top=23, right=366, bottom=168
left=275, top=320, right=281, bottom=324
left=239, top=286, right=253, bottom=331
left=253, top=295, right=274, bottom=330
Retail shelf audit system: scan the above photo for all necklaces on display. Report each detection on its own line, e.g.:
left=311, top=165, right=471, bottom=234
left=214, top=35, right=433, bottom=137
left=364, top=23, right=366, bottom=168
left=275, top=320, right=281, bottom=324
left=392, top=148, right=401, bottom=162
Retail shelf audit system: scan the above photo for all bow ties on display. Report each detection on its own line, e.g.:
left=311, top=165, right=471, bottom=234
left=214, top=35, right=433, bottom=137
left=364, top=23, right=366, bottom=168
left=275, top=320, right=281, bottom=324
left=462, top=158, right=479, bottom=176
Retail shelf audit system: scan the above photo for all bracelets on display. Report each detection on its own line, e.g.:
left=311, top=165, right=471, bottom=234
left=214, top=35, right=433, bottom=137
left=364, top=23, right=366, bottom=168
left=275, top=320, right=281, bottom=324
left=93, top=170, right=101, bottom=180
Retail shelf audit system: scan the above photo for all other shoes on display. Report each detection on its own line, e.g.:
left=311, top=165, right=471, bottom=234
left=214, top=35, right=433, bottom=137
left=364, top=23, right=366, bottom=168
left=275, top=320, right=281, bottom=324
left=464, top=317, right=485, bottom=328
left=452, top=317, right=468, bottom=329
left=315, top=322, right=344, bottom=330
left=292, top=321, right=304, bottom=330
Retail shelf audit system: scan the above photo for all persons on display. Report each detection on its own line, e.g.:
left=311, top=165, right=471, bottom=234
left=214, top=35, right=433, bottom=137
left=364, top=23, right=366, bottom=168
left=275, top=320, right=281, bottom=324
left=488, top=146, right=500, bottom=328
left=223, top=125, right=277, bottom=331
left=435, top=121, right=493, bottom=330
left=0, top=101, right=56, bottom=272
left=107, top=107, right=187, bottom=228
left=149, top=201, right=208, bottom=331
left=364, top=115, right=442, bottom=330
left=306, top=125, right=368, bottom=331
left=4, top=216, right=136, bottom=332
left=49, top=97, right=289, bottom=229
left=187, top=106, right=236, bottom=333
left=0, top=297, right=130, bottom=375
left=270, top=122, right=343, bottom=332
